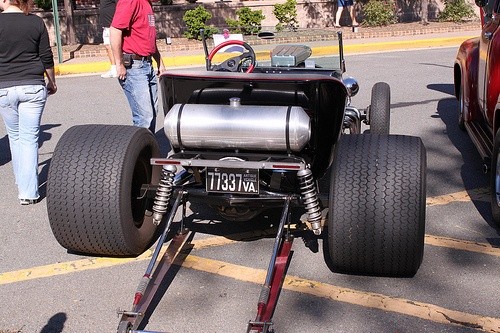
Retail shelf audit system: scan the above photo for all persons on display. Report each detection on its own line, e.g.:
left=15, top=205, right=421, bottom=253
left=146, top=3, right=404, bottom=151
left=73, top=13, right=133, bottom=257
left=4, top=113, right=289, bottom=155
left=0, top=0, right=57, bottom=204
left=110, top=0, right=166, bottom=133
left=335, top=0, right=360, bottom=28
left=100, top=0, right=119, bottom=79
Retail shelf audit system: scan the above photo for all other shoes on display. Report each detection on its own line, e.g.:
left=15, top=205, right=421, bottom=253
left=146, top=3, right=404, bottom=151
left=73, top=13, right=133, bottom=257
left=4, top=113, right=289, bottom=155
left=101, top=68, right=117, bottom=77
left=21, top=199, right=38, bottom=204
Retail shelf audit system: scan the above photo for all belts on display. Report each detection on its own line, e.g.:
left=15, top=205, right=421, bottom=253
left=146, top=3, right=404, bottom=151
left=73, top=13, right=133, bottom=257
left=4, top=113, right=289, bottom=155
left=132, top=54, right=151, bottom=60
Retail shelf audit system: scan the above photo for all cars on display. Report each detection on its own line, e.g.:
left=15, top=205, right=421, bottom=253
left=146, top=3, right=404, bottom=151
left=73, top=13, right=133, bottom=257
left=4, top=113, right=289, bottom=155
left=453, top=0, right=500, bottom=226
